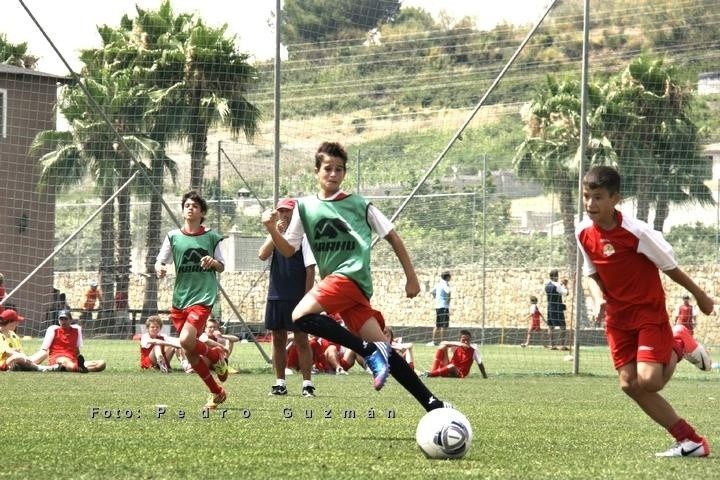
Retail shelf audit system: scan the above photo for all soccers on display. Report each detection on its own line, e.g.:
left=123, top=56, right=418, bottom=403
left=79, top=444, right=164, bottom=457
left=416, top=407, right=472, bottom=460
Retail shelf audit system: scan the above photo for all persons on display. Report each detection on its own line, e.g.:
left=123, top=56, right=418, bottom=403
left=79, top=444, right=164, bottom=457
left=575, top=167, right=714, bottom=456
left=0, top=268, right=698, bottom=379
left=258, top=199, right=319, bottom=398
left=152, top=191, right=231, bottom=411
left=263, top=143, right=454, bottom=412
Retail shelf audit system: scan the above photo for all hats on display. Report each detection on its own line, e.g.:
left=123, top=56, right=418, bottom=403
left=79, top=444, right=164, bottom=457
left=57, top=309, right=72, bottom=319
left=276, top=198, right=295, bottom=210
left=91, top=282, right=99, bottom=288
left=0, top=309, right=25, bottom=321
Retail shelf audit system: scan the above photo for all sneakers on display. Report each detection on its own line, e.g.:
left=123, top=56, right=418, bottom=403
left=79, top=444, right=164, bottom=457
left=671, top=325, right=710, bottom=371
left=417, top=371, right=429, bottom=378
left=156, top=354, right=239, bottom=382
left=302, top=385, right=316, bottom=398
left=204, top=386, right=226, bottom=409
left=284, top=364, right=349, bottom=376
left=76, top=355, right=88, bottom=373
left=43, top=363, right=62, bottom=372
left=655, top=435, right=708, bottom=456
left=365, top=340, right=392, bottom=391
left=268, top=385, right=288, bottom=396
